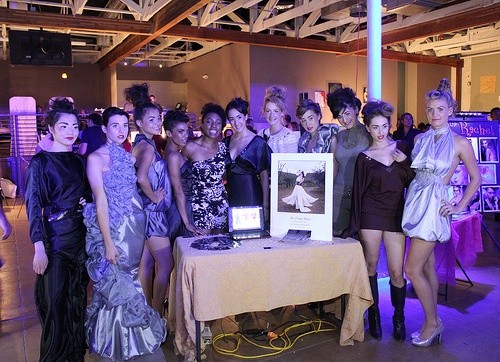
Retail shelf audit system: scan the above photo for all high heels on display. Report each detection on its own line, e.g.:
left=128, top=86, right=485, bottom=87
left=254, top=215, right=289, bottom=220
left=411, top=324, right=444, bottom=347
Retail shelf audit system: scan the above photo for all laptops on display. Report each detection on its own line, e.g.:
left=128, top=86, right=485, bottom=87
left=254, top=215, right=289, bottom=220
left=227, top=205, right=271, bottom=241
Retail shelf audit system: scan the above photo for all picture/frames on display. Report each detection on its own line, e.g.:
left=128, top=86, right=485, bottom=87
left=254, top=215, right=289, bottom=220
left=450, top=184, right=480, bottom=214
left=267, top=149, right=336, bottom=243
left=464, top=137, right=478, bottom=160
left=476, top=163, right=497, bottom=185
left=481, top=185, right=500, bottom=212
left=449, top=164, right=471, bottom=185
left=478, top=137, right=500, bottom=164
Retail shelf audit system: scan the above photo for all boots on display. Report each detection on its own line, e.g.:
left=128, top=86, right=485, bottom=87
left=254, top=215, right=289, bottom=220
left=366, top=272, right=383, bottom=339
left=389, top=278, right=409, bottom=342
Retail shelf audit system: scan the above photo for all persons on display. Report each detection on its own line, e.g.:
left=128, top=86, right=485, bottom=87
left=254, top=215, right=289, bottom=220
left=352, top=99, right=416, bottom=344
left=388, top=91, right=500, bottom=192
left=27, top=99, right=95, bottom=362
left=37, top=104, right=45, bottom=123
left=254, top=83, right=302, bottom=211
left=82, top=106, right=168, bottom=362
left=327, top=86, right=375, bottom=328
left=220, top=94, right=274, bottom=233
left=32, top=75, right=315, bottom=158
left=296, top=98, right=341, bottom=189
left=447, top=139, right=500, bottom=212
left=0, top=184, right=14, bottom=276
left=281, top=168, right=320, bottom=213
left=181, top=102, right=235, bottom=236
left=400, top=76, right=483, bottom=348
left=163, top=108, right=208, bottom=252
left=123, top=80, right=175, bottom=326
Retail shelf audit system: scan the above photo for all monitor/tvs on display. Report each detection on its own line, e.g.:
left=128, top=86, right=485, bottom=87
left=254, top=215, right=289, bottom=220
left=7, top=29, right=74, bottom=69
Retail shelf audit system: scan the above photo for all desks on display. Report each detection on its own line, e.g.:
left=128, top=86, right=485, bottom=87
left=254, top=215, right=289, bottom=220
left=166, top=226, right=374, bottom=361
